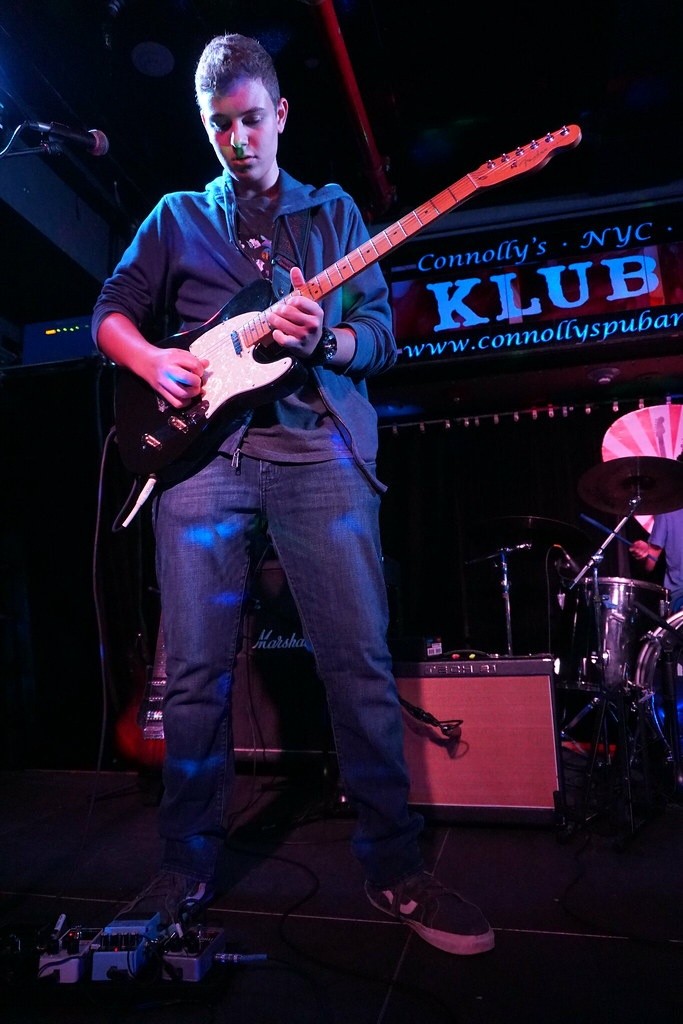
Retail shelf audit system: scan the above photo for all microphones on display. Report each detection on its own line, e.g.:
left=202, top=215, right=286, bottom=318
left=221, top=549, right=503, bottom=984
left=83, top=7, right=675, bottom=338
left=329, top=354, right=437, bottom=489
left=561, top=548, right=584, bottom=579
left=442, top=721, right=461, bottom=738
left=245, top=597, right=261, bottom=610
left=26, top=120, right=109, bottom=156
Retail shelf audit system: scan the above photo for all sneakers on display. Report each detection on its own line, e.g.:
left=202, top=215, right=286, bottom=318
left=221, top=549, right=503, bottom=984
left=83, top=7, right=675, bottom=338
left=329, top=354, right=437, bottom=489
left=115, top=869, right=216, bottom=935
left=364, top=872, right=494, bottom=956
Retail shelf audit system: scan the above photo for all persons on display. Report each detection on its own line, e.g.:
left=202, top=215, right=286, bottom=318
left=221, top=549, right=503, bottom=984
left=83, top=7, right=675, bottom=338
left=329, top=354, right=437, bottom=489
left=91, top=32, right=497, bottom=957
left=628, top=509, right=683, bottom=614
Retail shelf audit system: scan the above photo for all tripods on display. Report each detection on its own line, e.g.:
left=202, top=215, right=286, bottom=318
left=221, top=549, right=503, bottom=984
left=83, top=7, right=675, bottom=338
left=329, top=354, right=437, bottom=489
left=563, top=491, right=683, bottom=849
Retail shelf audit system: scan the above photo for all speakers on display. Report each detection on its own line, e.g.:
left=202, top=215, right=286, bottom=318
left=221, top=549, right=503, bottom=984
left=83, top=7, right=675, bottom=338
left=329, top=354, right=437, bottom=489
left=228, top=559, right=339, bottom=763
left=388, top=657, right=566, bottom=827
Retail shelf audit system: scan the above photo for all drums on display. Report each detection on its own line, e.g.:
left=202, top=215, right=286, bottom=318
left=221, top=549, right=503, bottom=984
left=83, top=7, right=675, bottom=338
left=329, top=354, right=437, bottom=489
left=635, top=609, right=683, bottom=790
left=564, top=577, right=671, bottom=695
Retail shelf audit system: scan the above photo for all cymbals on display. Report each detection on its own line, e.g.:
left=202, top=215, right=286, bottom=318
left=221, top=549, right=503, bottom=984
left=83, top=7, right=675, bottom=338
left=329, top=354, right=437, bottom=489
left=459, top=515, right=591, bottom=561
left=578, top=456, right=683, bottom=516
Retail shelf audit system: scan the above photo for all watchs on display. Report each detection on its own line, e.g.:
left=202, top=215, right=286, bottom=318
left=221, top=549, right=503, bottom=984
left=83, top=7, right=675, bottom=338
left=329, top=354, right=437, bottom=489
left=306, top=326, right=338, bottom=365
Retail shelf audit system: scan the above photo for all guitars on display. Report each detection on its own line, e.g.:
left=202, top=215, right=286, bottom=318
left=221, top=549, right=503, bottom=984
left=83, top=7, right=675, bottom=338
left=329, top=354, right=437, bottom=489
left=112, top=122, right=583, bottom=481
left=108, top=604, right=167, bottom=768
